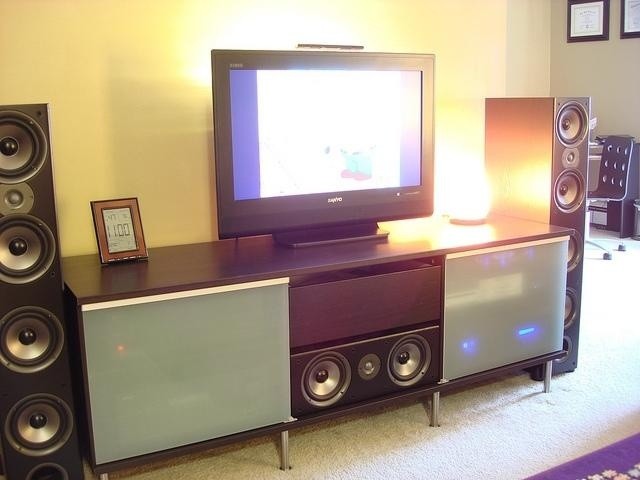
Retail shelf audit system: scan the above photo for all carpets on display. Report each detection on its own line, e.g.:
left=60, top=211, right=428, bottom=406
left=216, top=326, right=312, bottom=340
left=522, top=430, right=640, bottom=480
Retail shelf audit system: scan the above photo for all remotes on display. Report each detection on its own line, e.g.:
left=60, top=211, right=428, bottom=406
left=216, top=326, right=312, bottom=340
left=298, top=43, right=365, bottom=50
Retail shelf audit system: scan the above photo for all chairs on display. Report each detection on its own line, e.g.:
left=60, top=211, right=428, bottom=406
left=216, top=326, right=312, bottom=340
left=586, top=135, right=633, bottom=261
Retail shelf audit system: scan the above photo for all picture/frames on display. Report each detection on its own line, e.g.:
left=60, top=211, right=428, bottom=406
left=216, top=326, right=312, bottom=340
left=620, top=0, right=640, bottom=39
left=567, top=0, right=609, bottom=42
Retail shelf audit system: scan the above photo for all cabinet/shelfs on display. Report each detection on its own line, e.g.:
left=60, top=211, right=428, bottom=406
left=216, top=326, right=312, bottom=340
left=57, top=212, right=574, bottom=480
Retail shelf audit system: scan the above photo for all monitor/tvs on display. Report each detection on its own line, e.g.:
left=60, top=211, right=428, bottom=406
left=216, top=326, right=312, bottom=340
left=210, top=47, right=435, bottom=250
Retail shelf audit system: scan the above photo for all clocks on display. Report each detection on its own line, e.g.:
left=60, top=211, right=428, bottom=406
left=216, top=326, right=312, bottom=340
left=90, top=197, right=148, bottom=265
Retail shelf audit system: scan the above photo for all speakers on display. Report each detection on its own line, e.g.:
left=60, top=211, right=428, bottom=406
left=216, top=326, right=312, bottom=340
left=482, top=94, right=591, bottom=382
left=290, top=322, right=441, bottom=418
left=0, top=103, right=86, bottom=480
